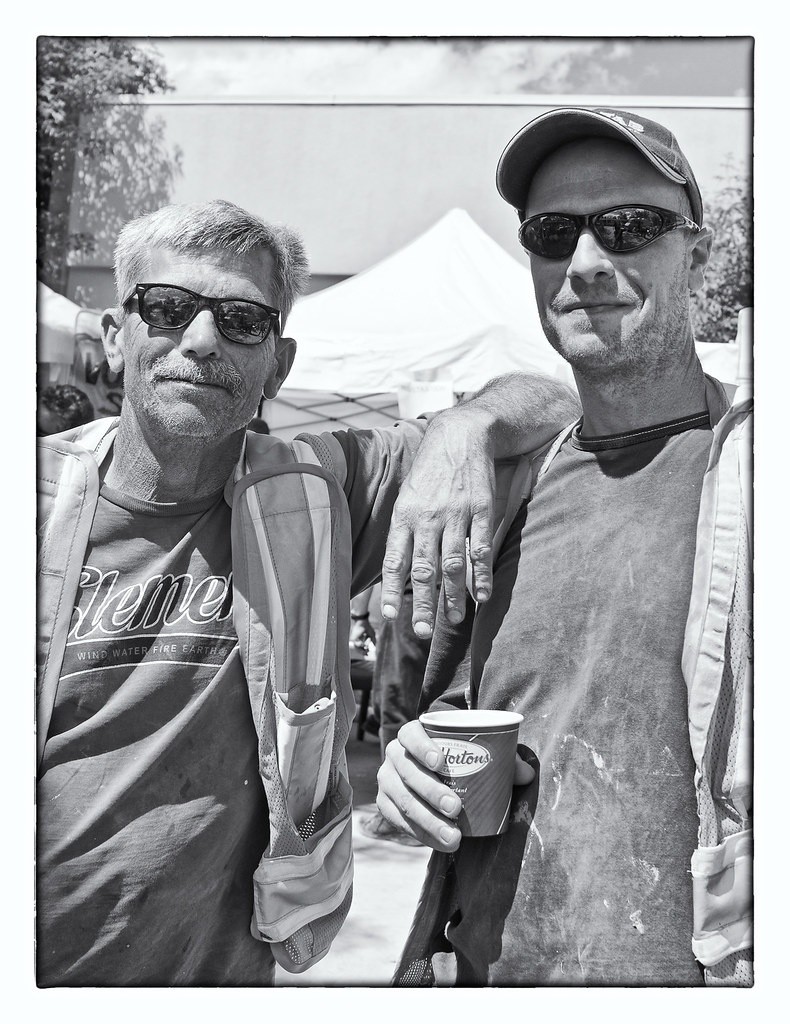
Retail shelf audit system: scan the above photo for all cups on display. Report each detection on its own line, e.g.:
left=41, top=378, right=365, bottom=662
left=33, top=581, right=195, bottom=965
left=420, top=710, right=524, bottom=837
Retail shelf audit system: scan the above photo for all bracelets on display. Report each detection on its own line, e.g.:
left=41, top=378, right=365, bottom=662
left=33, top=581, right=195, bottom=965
left=351, top=612, right=369, bottom=620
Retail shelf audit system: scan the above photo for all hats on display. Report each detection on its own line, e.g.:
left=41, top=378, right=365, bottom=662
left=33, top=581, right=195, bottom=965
left=496, top=108, right=703, bottom=229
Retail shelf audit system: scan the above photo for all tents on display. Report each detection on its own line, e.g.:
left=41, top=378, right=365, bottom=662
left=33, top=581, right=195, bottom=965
left=37, top=279, right=105, bottom=367
left=249, top=206, right=738, bottom=444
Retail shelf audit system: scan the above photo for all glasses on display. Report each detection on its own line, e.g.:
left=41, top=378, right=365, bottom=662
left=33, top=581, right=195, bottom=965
left=518, top=204, right=700, bottom=260
left=123, top=283, right=282, bottom=345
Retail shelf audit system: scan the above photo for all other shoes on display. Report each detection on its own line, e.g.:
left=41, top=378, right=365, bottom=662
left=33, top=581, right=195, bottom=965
left=363, top=715, right=379, bottom=737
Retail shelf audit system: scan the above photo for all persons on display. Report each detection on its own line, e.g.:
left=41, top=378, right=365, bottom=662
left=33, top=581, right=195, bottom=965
left=375, top=104, right=753, bottom=988
left=343, top=549, right=444, bottom=848
left=37, top=200, right=586, bottom=989
